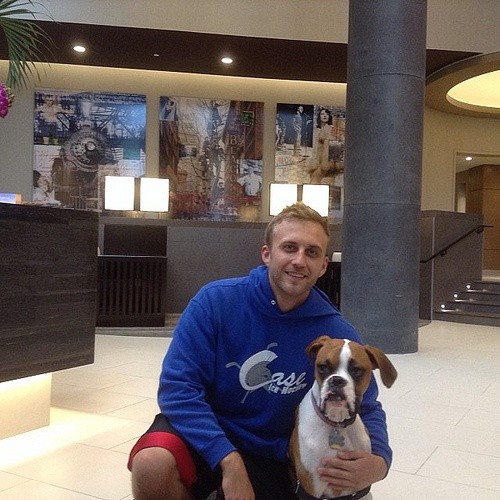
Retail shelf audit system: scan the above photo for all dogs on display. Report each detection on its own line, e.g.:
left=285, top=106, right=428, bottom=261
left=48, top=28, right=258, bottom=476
left=288, top=334, right=398, bottom=500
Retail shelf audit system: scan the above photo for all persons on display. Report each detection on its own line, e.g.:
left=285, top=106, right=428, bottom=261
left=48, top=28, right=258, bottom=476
left=127, top=200, right=394, bottom=500
left=33, top=91, right=347, bottom=222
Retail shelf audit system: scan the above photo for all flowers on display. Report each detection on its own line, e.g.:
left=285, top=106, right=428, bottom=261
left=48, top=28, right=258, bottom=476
left=0, top=0, right=63, bottom=119
